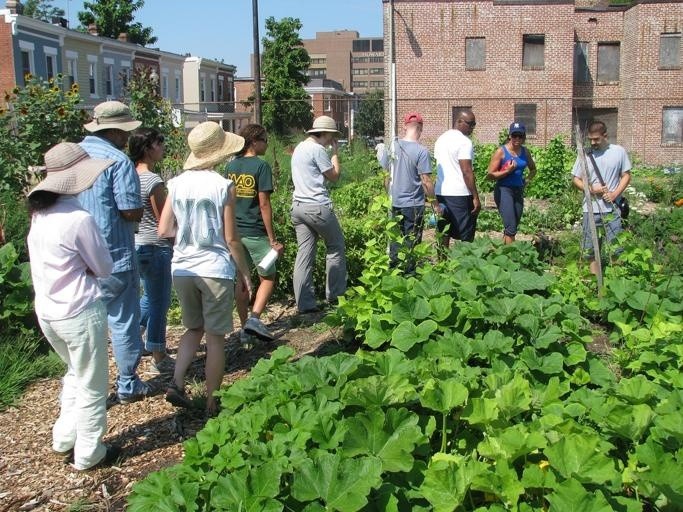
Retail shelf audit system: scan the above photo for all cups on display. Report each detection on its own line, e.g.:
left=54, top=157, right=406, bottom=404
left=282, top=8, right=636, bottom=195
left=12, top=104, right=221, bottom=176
left=617, top=195, right=629, bottom=220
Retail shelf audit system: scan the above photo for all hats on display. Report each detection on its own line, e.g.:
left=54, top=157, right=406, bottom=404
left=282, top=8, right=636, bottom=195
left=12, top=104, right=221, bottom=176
left=402, top=111, right=424, bottom=126
left=508, top=121, right=528, bottom=136
left=181, top=120, right=245, bottom=171
left=82, top=98, right=144, bottom=134
left=25, top=139, right=119, bottom=200
left=305, top=114, right=342, bottom=136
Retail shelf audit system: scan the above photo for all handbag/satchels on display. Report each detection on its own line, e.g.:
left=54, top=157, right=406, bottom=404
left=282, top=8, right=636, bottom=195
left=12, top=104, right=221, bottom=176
left=616, top=196, right=630, bottom=220
left=498, top=158, right=518, bottom=177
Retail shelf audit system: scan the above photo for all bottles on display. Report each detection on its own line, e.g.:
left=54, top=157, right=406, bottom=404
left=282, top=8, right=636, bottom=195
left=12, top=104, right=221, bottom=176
left=429, top=203, right=446, bottom=226
left=257, top=248, right=278, bottom=272
left=500, top=157, right=513, bottom=174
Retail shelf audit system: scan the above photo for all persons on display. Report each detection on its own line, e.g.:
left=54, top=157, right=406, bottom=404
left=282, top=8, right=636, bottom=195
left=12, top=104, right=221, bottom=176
left=488, top=122, right=537, bottom=245
left=223, top=124, right=285, bottom=352
left=570, top=121, right=633, bottom=275
left=434, top=110, right=481, bottom=261
left=74, top=100, right=159, bottom=405
left=374, top=139, right=388, bottom=170
left=27, top=139, right=120, bottom=470
left=289, top=116, right=352, bottom=312
left=124, top=127, right=183, bottom=376
left=389, top=112, right=445, bottom=279
left=157, top=120, right=257, bottom=423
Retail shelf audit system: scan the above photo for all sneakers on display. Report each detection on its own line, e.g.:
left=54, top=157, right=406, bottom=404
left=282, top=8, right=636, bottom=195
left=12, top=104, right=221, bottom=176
left=201, top=406, right=220, bottom=424
left=164, top=380, right=196, bottom=411
left=243, top=317, right=276, bottom=342
left=115, top=378, right=163, bottom=405
left=239, top=328, right=252, bottom=344
left=300, top=304, right=323, bottom=314
left=148, top=353, right=192, bottom=374
left=51, top=436, right=119, bottom=471
left=141, top=341, right=151, bottom=356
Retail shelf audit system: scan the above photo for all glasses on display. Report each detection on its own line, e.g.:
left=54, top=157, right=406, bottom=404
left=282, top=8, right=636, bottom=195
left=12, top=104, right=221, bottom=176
left=256, top=137, right=268, bottom=143
left=459, top=118, right=477, bottom=128
